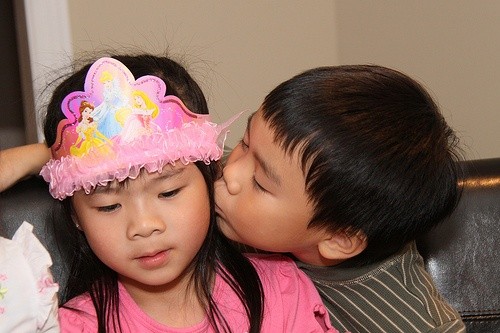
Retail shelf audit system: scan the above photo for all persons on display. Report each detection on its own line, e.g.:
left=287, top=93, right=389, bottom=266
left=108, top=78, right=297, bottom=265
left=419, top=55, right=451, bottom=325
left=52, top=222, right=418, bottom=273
left=40, top=50, right=341, bottom=333
left=1, top=64, right=469, bottom=332
